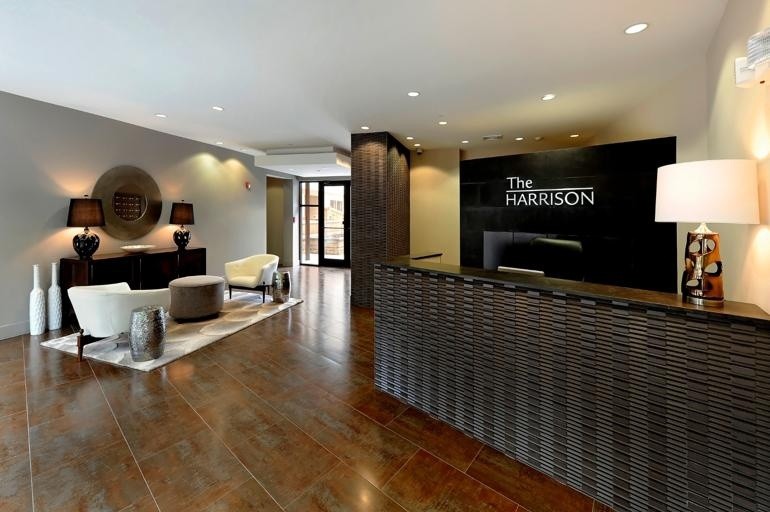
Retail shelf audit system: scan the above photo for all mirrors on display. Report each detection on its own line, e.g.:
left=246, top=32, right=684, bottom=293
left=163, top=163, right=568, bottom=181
left=90, top=164, right=163, bottom=242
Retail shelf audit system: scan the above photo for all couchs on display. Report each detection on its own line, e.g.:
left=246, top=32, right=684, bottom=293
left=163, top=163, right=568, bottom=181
left=62, top=278, right=177, bottom=368
left=223, top=253, right=279, bottom=304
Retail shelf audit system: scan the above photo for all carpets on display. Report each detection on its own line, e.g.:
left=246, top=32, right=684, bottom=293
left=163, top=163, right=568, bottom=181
left=37, top=288, right=306, bottom=375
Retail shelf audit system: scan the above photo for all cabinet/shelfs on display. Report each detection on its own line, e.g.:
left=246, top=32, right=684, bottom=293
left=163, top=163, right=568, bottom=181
left=58, top=245, right=208, bottom=320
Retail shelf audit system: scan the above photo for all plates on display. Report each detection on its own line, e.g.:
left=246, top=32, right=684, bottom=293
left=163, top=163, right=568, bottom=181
left=119, top=244, right=156, bottom=253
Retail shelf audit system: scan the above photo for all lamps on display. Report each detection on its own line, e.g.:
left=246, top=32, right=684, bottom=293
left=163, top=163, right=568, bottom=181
left=65, top=193, right=107, bottom=260
left=166, top=195, right=199, bottom=248
left=649, top=156, right=766, bottom=309
left=744, top=27, right=769, bottom=69
left=733, top=55, right=756, bottom=88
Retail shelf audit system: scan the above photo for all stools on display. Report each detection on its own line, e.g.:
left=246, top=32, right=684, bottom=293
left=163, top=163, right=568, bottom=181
left=125, top=303, right=166, bottom=364
left=269, top=270, right=292, bottom=304
left=165, top=273, right=224, bottom=325
